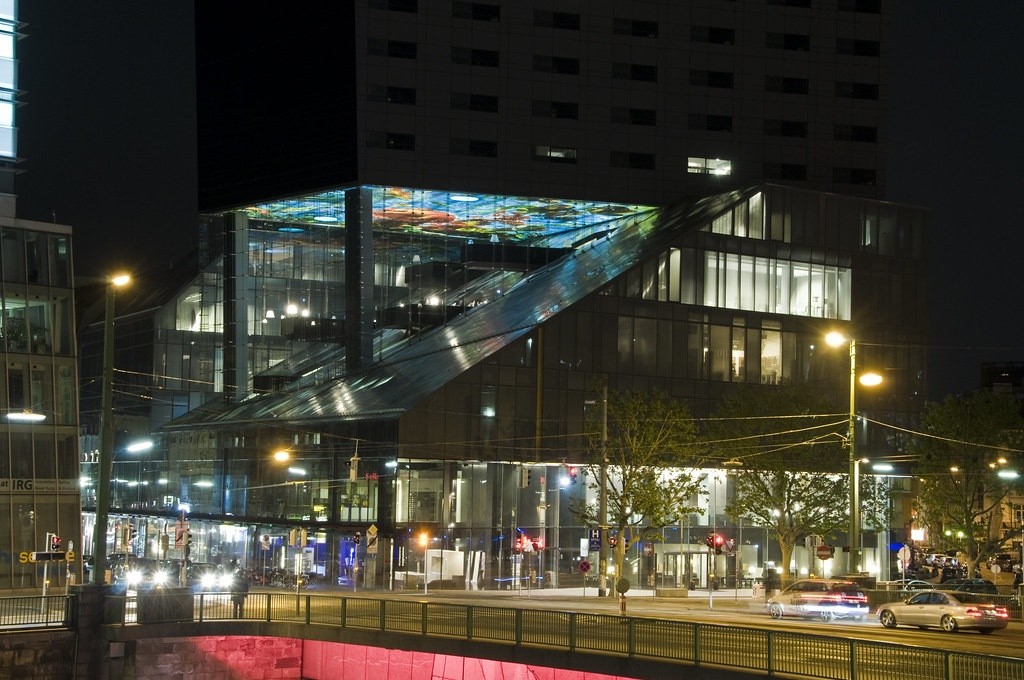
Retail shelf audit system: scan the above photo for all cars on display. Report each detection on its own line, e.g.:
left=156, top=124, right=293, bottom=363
left=109, top=557, right=225, bottom=591
left=82, top=555, right=94, bottom=567
left=767, top=579, right=869, bottom=623
left=876, top=590, right=1008, bottom=634
left=938, top=578, right=998, bottom=594
left=896, top=579, right=937, bottom=598
left=105, top=553, right=136, bottom=569
left=914, top=547, right=982, bottom=583
left=1013, top=572, right=1023, bottom=585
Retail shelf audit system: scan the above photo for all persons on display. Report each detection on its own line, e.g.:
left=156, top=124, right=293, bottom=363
left=231, top=570, right=248, bottom=619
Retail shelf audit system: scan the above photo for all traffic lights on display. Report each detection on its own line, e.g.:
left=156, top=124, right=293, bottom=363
left=715, top=536, right=723, bottom=555
left=523, top=469, right=531, bottom=486
left=262, top=534, right=269, bottom=550
left=183, top=532, right=192, bottom=545
left=622, top=538, right=630, bottom=554
left=570, top=466, right=577, bottom=485
left=51, top=535, right=61, bottom=550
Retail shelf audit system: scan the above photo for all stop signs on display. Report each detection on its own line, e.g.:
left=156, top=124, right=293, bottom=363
left=817, top=546, right=830, bottom=560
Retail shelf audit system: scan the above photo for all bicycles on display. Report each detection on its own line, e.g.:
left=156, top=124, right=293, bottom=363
left=267, top=568, right=309, bottom=589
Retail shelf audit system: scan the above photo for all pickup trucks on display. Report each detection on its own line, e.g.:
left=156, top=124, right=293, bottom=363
left=986, top=553, right=1017, bottom=571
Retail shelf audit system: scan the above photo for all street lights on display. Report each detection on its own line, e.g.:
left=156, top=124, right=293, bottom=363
left=584, top=386, right=606, bottom=597
left=999, top=458, right=1013, bottom=528
left=709, top=476, right=722, bottom=609
left=420, top=535, right=428, bottom=595
left=827, top=332, right=882, bottom=574
left=89, top=273, right=131, bottom=584
left=854, top=458, right=869, bottom=547
